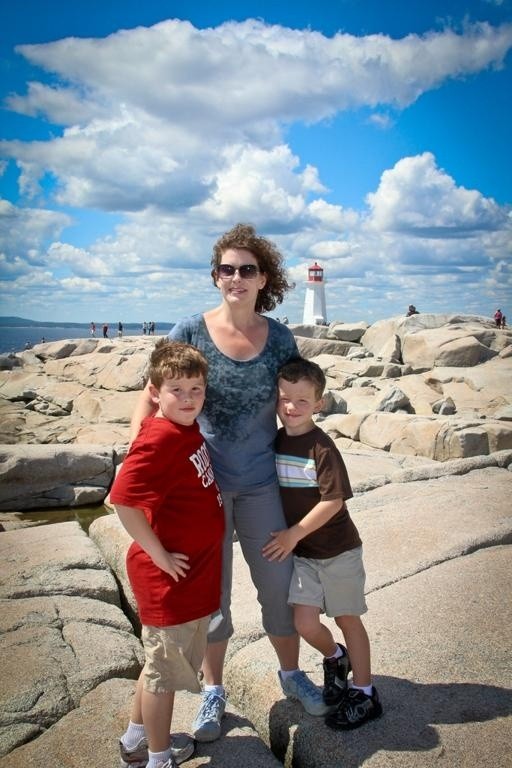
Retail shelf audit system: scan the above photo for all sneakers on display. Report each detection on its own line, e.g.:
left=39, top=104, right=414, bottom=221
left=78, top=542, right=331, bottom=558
left=322, top=642, right=353, bottom=707
left=192, top=688, right=226, bottom=742
left=277, top=668, right=331, bottom=716
left=117, top=731, right=195, bottom=768
left=324, top=685, right=383, bottom=731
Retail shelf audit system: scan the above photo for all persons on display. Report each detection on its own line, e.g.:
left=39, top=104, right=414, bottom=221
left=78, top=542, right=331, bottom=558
left=108, top=340, right=227, bottom=768
left=405, top=304, right=420, bottom=317
left=493, top=308, right=502, bottom=329
left=90, top=321, right=96, bottom=338
left=102, top=323, right=108, bottom=338
left=142, top=321, right=155, bottom=336
left=259, top=357, right=381, bottom=731
left=501, top=316, right=506, bottom=329
left=118, top=321, right=122, bottom=337
left=128, top=223, right=329, bottom=744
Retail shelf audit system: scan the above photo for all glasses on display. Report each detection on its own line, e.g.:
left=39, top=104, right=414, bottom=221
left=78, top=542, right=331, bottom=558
left=214, top=264, right=264, bottom=279
